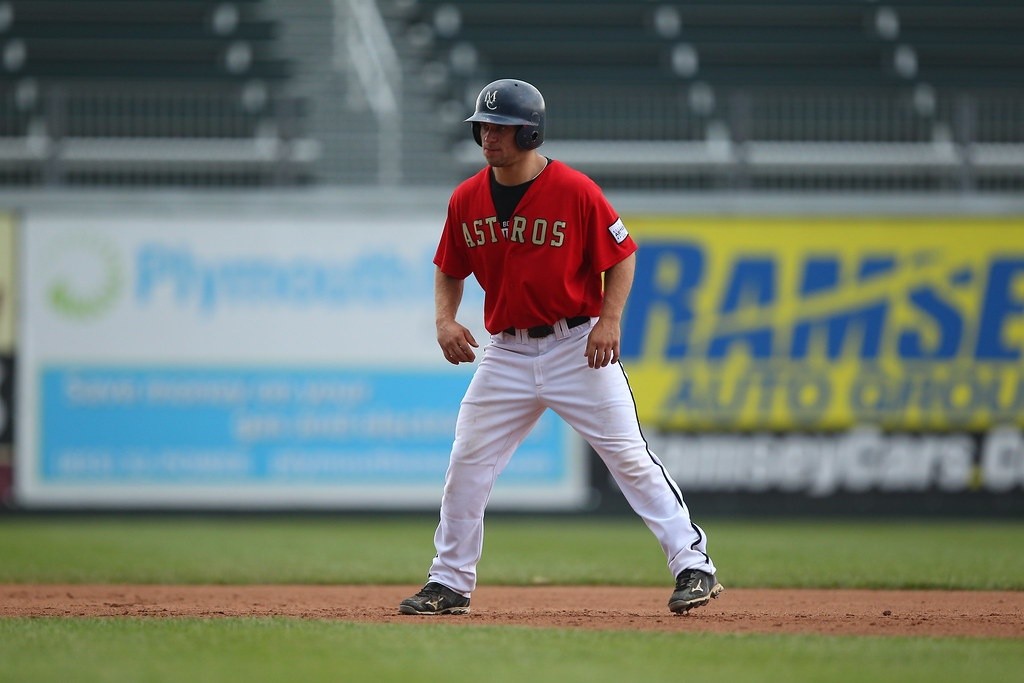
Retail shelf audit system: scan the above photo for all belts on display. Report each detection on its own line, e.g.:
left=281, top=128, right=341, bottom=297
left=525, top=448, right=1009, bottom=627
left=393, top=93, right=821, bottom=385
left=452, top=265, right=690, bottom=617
left=504, top=314, right=591, bottom=338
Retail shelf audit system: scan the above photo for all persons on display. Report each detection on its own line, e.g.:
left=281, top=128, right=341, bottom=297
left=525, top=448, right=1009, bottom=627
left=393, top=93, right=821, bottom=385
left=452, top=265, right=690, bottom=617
left=398, top=78, right=724, bottom=616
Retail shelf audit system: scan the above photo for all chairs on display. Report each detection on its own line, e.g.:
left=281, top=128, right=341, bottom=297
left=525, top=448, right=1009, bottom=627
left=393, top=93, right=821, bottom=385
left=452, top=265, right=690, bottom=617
left=0, top=0, right=1024, bottom=196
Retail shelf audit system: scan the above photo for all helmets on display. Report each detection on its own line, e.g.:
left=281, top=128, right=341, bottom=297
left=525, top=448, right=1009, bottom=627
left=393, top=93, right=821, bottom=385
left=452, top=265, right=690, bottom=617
left=463, top=79, right=545, bottom=151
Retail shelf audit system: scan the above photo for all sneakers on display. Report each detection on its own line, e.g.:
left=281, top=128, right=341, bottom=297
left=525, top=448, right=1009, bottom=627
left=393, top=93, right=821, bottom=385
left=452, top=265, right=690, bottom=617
left=400, top=583, right=473, bottom=615
left=668, top=568, right=724, bottom=611
